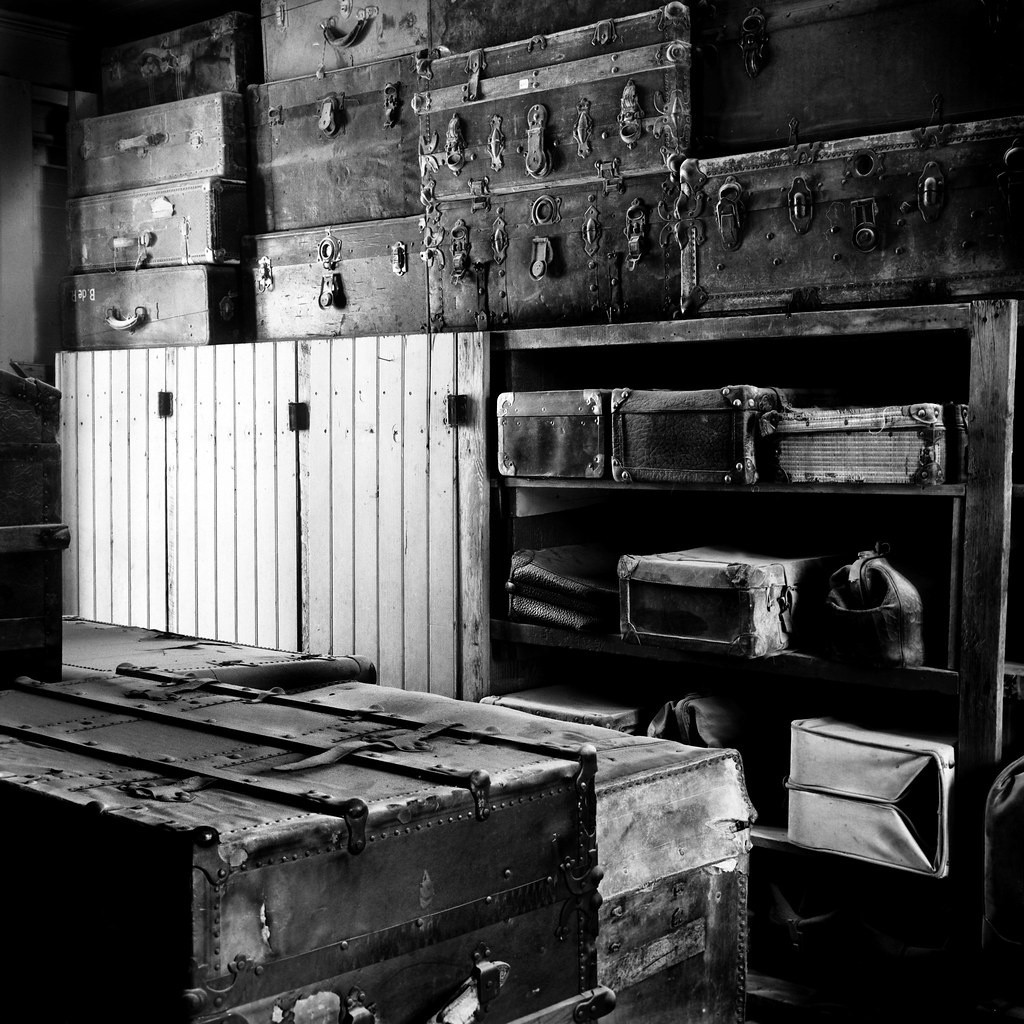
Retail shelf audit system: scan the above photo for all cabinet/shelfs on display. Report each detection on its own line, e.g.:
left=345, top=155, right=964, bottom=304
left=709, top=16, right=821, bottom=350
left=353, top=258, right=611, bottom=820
left=499, top=296, right=1021, bottom=983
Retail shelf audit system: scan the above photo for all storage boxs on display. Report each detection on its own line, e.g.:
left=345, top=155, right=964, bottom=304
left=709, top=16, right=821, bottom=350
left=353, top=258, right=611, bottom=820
left=496, top=390, right=968, bottom=487
left=618, top=540, right=837, bottom=660
left=780, top=707, right=959, bottom=880
left=0, top=369, right=757, bottom=1024
left=243, top=1, right=1024, bottom=333
